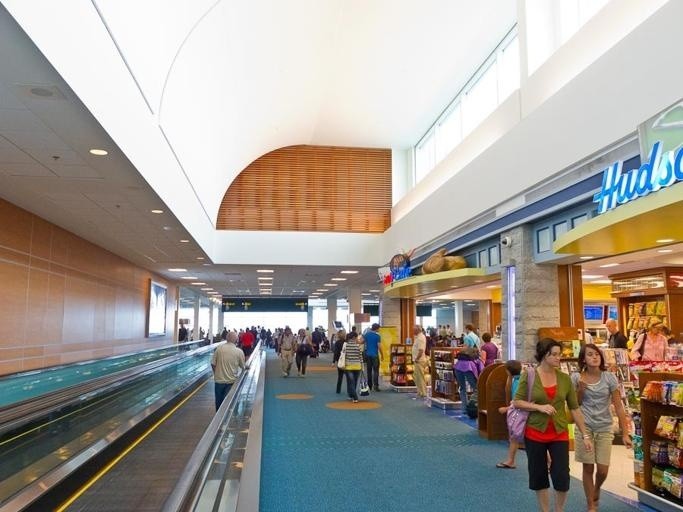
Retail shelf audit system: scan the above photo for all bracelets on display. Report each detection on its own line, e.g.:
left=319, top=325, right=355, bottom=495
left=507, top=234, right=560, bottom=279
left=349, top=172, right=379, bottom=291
left=582, top=435, right=588, bottom=438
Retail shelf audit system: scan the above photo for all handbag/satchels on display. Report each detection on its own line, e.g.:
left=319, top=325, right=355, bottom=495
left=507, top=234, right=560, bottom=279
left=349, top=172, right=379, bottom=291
left=505, top=367, right=536, bottom=442
left=338, top=342, right=347, bottom=369
left=358, top=370, right=371, bottom=397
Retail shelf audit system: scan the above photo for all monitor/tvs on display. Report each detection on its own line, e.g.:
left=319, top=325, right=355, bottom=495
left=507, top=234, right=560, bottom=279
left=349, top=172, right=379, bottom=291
left=607, top=305, right=618, bottom=324
left=583, top=304, right=604, bottom=324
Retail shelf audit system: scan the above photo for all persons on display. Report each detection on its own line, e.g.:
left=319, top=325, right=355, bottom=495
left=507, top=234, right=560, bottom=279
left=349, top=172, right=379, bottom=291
left=631, top=317, right=668, bottom=361
left=514, top=338, right=593, bottom=512
left=569, top=344, right=633, bottom=512
left=661, top=325, right=678, bottom=344
left=496, top=360, right=521, bottom=469
left=605, top=318, right=628, bottom=349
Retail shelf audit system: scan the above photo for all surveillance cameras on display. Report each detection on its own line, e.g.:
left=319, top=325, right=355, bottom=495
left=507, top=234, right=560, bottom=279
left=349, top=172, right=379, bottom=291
left=501, top=236, right=511, bottom=247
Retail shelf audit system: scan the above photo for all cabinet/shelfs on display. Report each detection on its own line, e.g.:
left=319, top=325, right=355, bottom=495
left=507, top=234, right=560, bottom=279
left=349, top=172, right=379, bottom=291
left=391, top=344, right=431, bottom=393
left=476, top=363, right=509, bottom=440
left=628, top=371, right=683, bottom=512
left=430, top=347, right=464, bottom=409
left=608, top=266, right=683, bottom=343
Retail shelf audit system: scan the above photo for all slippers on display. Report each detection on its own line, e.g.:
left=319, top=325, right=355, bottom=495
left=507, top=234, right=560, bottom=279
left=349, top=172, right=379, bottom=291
left=496, top=462, right=517, bottom=469
